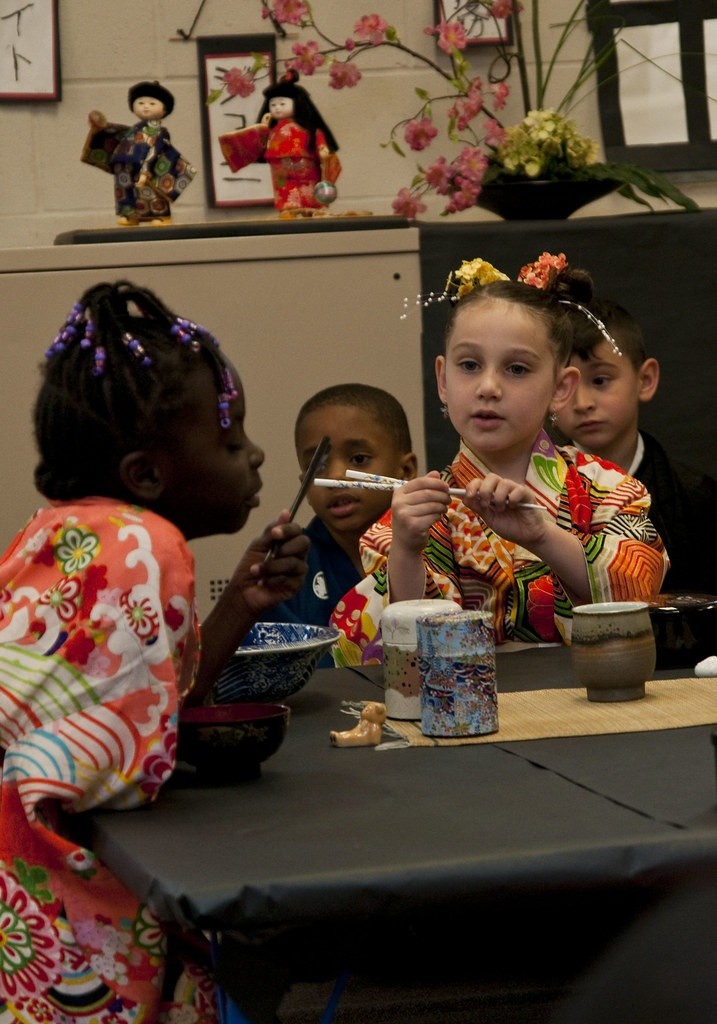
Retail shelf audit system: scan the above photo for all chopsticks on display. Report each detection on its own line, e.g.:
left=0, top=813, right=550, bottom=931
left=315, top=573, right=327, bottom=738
left=262, top=437, right=330, bottom=577
left=314, top=469, right=548, bottom=509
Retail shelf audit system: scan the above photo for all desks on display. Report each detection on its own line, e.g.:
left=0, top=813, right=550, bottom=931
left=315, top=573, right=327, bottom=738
left=69, top=617, right=717, bottom=1024
left=57, top=202, right=717, bottom=598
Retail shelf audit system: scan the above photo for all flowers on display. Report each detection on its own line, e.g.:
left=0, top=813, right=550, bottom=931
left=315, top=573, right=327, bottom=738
left=398, top=254, right=511, bottom=320
left=518, top=250, right=567, bottom=290
left=206, top=0, right=703, bottom=215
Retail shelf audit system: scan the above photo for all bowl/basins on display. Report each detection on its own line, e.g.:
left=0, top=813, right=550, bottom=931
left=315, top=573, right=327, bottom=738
left=199, top=623, right=342, bottom=702
left=627, top=595, right=716, bottom=670
left=163, top=703, right=289, bottom=790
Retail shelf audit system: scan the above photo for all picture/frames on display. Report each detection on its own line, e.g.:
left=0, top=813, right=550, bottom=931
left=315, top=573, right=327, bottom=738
left=429, top=0, right=518, bottom=50
left=197, top=34, right=284, bottom=205
left=0, top=0, right=62, bottom=103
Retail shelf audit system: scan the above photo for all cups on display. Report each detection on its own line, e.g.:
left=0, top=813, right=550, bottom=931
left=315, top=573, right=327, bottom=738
left=415, top=610, right=498, bottom=737
left=571, top=603, right=656, bottom=702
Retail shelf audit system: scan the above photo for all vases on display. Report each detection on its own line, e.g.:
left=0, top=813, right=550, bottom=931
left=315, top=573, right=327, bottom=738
left=450, top=171, right=627, bottom=221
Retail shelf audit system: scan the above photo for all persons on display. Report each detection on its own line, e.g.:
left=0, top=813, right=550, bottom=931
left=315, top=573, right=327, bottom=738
left=220, top=382, right=417, bottom=705
left=2, top=276, right=307, bottom=1023
left=550, top=294, right=716, bottom=674
left=80, top=79, right=196, bottom=224
left=219, top=67, right=342, bottom=214
left=325, top=259, right=668, bottom=670
